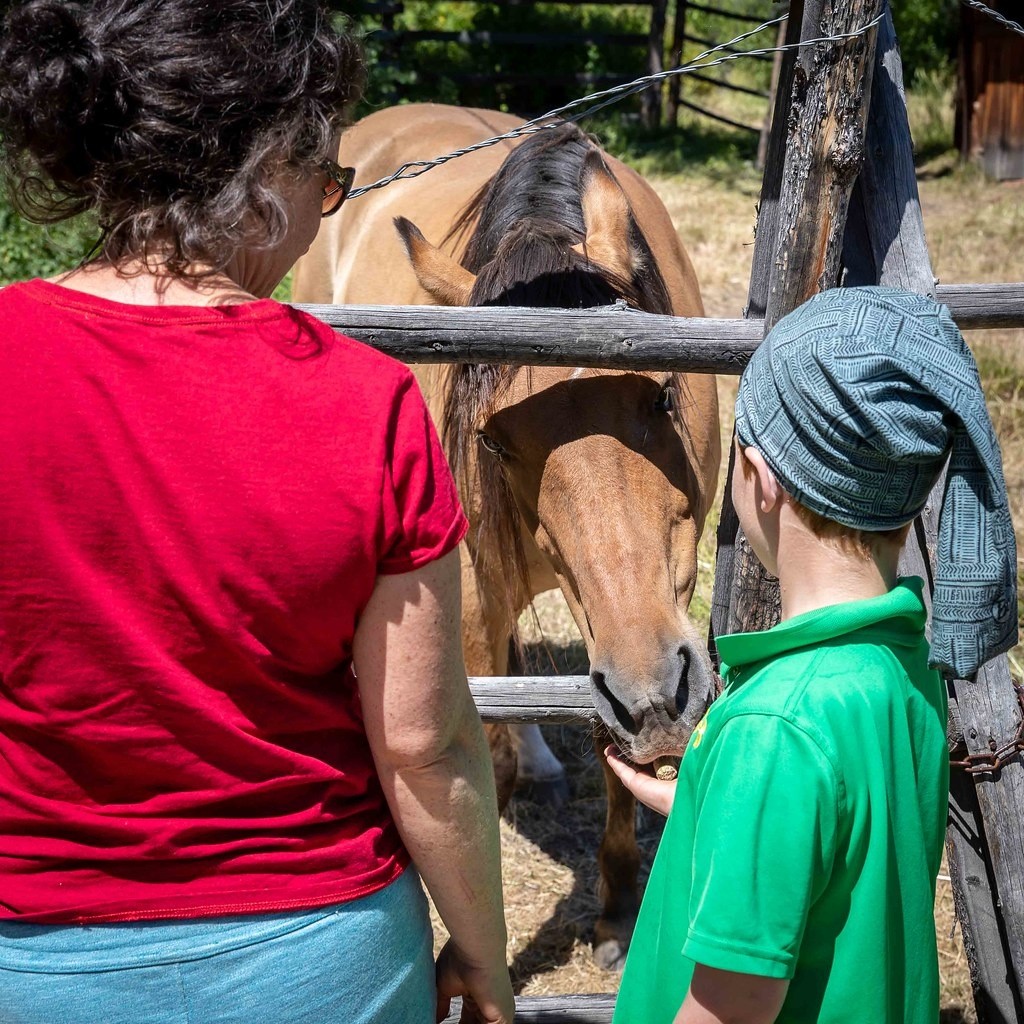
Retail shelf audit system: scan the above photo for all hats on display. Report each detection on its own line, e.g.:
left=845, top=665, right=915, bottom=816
left=734, top=284, right=1020, bottom=679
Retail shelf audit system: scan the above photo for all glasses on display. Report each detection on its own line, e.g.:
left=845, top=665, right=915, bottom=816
left=310, top=148, right=356, bottom=219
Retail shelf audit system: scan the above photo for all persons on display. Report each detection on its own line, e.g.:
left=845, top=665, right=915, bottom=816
left=1, top=0, right=520, bottom=1024
left=599, top=283, right=1016, bottom=1022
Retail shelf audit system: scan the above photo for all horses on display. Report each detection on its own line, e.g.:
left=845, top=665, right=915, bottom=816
left=288, top=104, right=722, bottom=973
